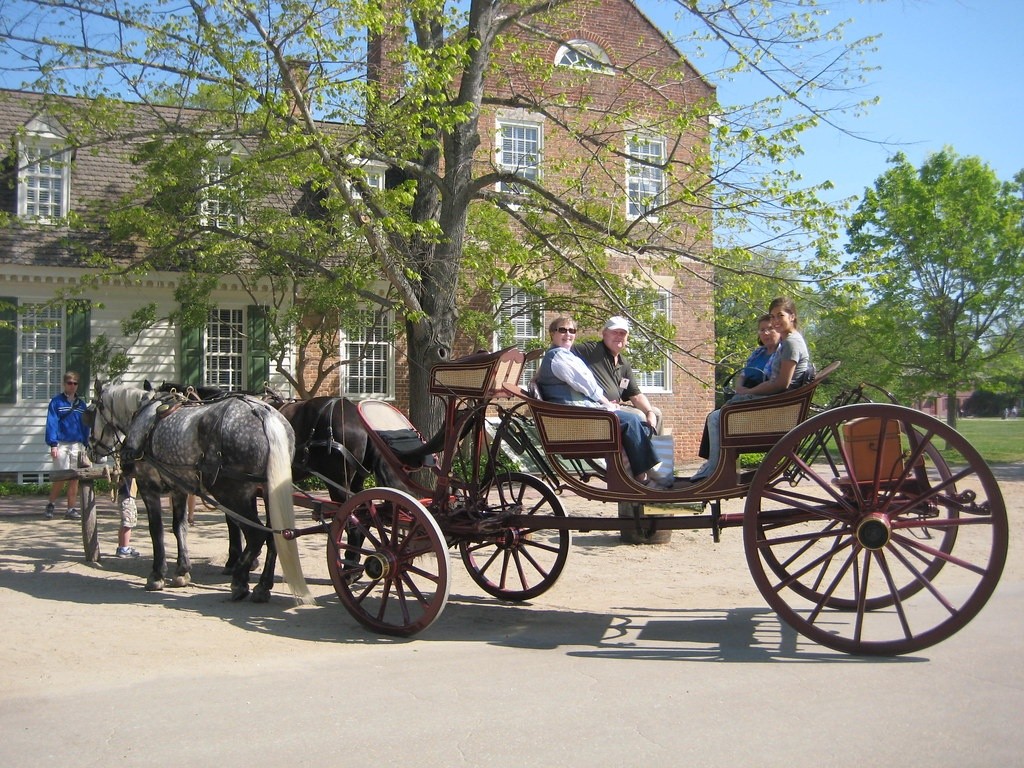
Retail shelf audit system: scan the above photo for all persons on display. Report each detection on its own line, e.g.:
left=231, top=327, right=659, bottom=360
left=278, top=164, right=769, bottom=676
left=45, top=370, right=90, bottom=520
left=532, top=317, right=664, bottom=438
left=690, top=297, right=809, bottom=482
left=116, top=474, right=140, bottom=559
left=537, top=316, right=675, bottom=487
left=1003, top=406, right=1017, bottom=418
left=169, top=492, right=195, bottom=533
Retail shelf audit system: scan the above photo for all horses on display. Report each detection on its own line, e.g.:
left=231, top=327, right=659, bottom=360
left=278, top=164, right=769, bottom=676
left=86, top=376, right=317, bottom=607
left=142, top=380, right=415, bottom=584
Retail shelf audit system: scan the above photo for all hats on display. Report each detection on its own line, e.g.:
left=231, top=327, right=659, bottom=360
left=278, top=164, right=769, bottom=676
left=604, top=316, right=629, bottom=334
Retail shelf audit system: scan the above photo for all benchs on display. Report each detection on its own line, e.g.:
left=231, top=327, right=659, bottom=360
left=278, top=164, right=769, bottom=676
left=428, top=343, right=527, bottom=399
left=718, top=360, right=841, bottom=454
left=502, top=382, right=623, bottom=460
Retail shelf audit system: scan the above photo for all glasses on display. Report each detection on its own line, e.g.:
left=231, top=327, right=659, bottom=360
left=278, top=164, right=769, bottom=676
left=66, top=382, right=78, bottom=385
left=556, top=328, right=577, bottom=334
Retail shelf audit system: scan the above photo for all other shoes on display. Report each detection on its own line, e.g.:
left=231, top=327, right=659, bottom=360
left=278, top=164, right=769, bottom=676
left=188, top=518, right=195, bottom=529
left=689, top=461, right=714, bottom=481
left=642, top=478, right=670, bottom=490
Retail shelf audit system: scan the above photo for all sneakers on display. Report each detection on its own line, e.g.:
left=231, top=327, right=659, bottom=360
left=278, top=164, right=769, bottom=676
left=65, top=509, right=82, bottom=520
left=116, top=547, right=140, bottom=559
left=45, top=502, right=55, bottom=518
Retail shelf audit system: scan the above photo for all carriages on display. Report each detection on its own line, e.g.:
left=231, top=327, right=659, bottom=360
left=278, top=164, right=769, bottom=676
left=86, top=345, right=1008, bottom=657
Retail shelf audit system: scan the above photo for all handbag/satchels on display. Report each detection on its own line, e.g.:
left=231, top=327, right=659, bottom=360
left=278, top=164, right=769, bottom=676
left=649, top=435, right=674, bottom=487
left=722, top=367, right=768, bottom=403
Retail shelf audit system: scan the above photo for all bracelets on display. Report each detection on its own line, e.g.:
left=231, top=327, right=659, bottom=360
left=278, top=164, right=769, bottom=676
left=647, top=410, right=653, bottom=416
